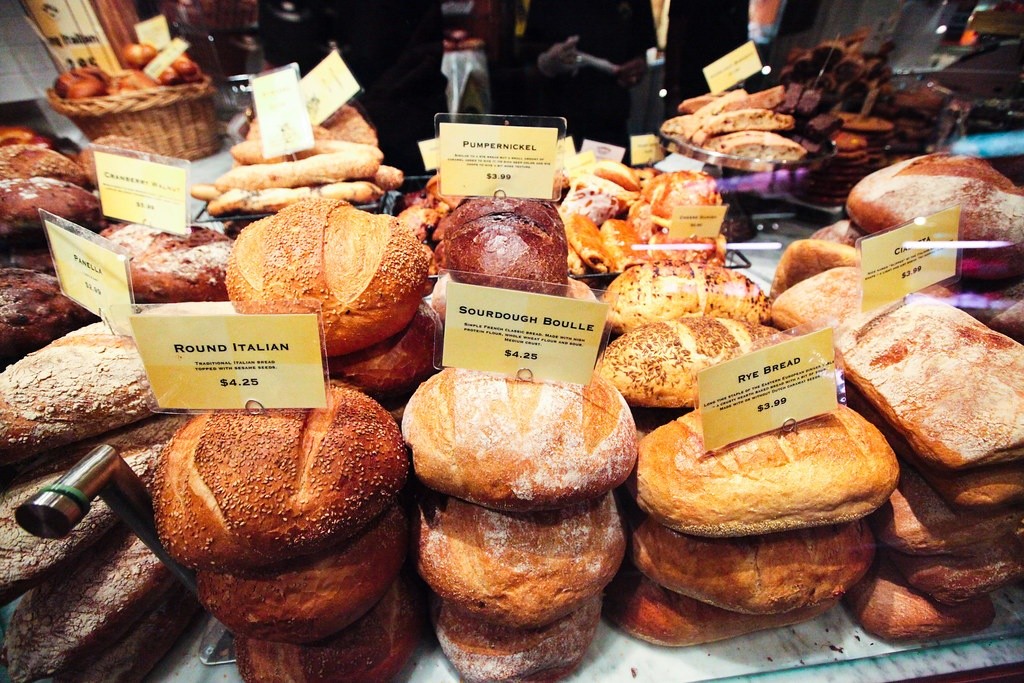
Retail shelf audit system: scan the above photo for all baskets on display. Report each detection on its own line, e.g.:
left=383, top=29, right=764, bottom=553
left=47, top=76, right=221, bottom=161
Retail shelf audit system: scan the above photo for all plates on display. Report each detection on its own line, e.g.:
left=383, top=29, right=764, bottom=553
left=784, top=193, right=845, bottom=215
left=664, top=124, right=838, bottom=176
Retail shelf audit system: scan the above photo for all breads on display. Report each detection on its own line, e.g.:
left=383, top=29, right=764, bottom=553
left=0, top=26, right=1024, bottom=683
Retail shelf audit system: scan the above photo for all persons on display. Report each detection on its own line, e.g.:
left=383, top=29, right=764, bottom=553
left=517, top=0, right=749, bottom=158
left=258, top=0, right=450, bottom=193
